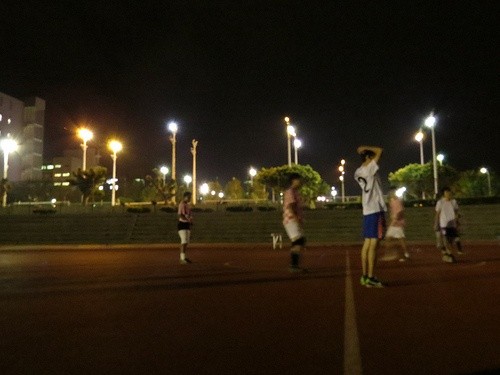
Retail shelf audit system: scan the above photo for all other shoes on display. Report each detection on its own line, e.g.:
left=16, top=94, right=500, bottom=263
left=399, top=253, right=409, bottom=261
left=445, top=251, right=454, bottom=261
left=180, top=258, right=192, bottom=264
left=290, top=267, right=304, bottom=272
left=383, top=255, right=398, bottom=261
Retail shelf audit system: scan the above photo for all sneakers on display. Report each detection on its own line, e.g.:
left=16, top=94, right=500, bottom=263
left=360, top=276, right=368, bottom=285
left=366, top=277, right=389, bottom=288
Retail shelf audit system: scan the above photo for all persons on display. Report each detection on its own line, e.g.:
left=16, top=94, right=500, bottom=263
left=282, top=173, right=308, bottom=273
left=176, top=192, right=194, bottom=265
left=434, top=187, right=463, bottom=263
left=381, top=186, right=411, bottom=263
left=354, top=146, right=389, bottom=288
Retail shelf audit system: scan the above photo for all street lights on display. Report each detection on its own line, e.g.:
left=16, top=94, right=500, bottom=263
left=77, top=129, right=93, bottom=202
left=480, top=168, right=490, bottom=189
left=169, top=122, right=178, bottom=203
left=415, top=132, right=426, bottom=200
left=109, top=139, right=121, bottom=206
left=337, top=159, right=345, bottom=204
left=161, top=166, right=169, bottom=186
left=425, top=116, right=438, bottom=195
left=1, top=139, right=18, bottom=207
left=184, top=175, right=192, bottom=190
left=285, top=116, right=302, bottom=169
left=436, top=153, right=444, bottom=166
